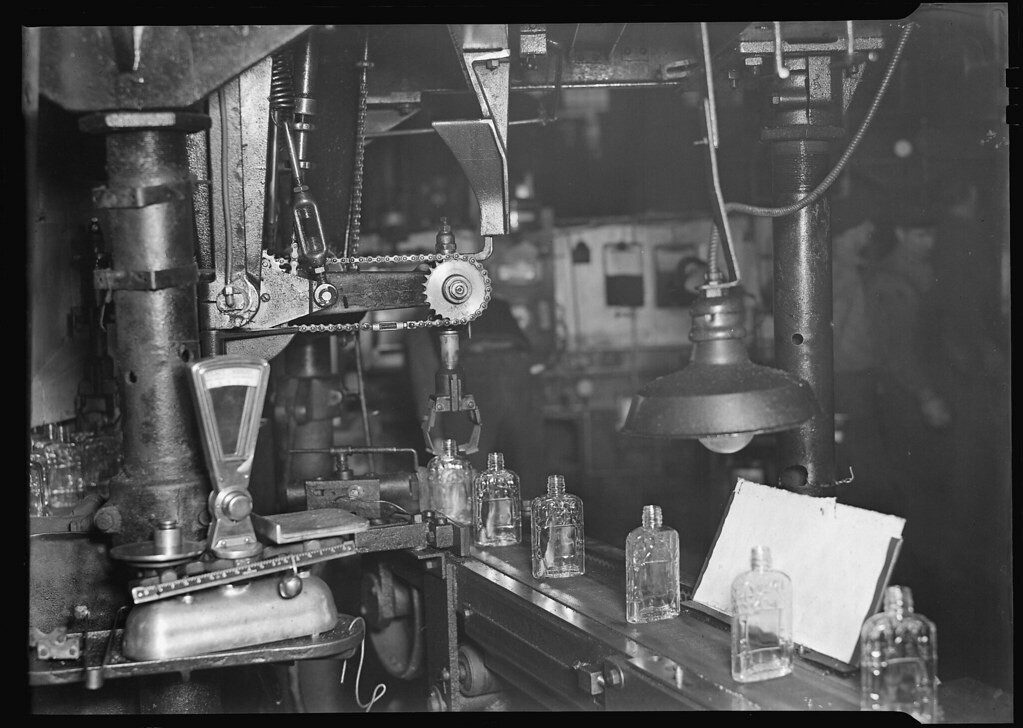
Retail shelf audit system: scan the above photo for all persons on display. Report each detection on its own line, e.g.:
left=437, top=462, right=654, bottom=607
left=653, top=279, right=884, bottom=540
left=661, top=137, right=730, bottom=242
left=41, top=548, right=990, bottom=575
left=410, top=297, right=545, bottom=499
left=833, top=205, right=953, bottom=428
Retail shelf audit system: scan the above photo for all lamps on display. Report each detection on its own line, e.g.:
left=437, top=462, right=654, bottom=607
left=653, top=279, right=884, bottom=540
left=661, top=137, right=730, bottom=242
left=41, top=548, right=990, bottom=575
left=620, top=18, right=922, bottom=453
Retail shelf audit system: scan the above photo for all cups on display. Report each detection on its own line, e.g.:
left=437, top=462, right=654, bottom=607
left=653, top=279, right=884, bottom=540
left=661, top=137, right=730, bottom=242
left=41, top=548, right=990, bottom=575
left=30, top=421, right=111, bottom=517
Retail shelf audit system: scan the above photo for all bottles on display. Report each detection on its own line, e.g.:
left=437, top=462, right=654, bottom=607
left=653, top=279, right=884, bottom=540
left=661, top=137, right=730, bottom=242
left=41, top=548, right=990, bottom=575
left=731, top=546, right=795, bottom=683
left=626, top=505, right=681, bottom=624
left=530, top=474, right=585, bottom=579
left=427, top=440, right=474, bottom=526
left=858, top=586, right=937, bottom=724
left=472, top=452, right=523, bottom=548
